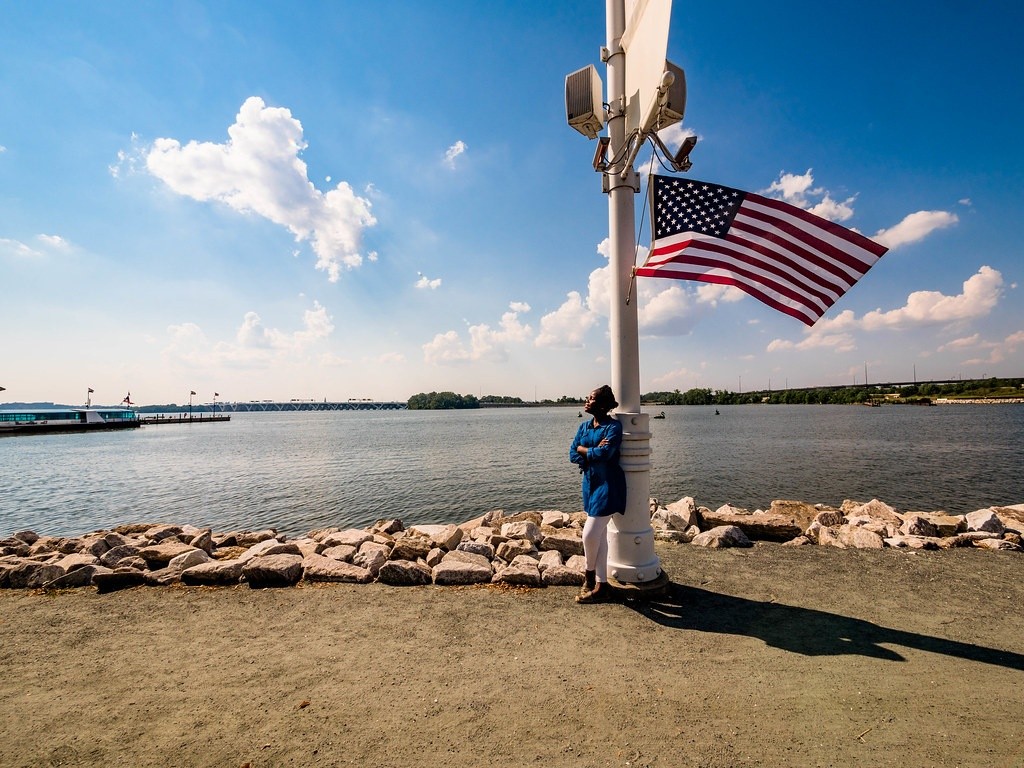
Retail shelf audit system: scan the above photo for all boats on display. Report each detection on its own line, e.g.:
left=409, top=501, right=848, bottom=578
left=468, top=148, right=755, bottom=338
left=0, top=408, right=140, bottom=435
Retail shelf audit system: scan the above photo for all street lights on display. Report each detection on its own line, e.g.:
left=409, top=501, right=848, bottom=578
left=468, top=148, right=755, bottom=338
left=982, top=374, right=987, bottom=399
left=864, top=361, right=867, bottom=386
left=912, top=363, right=915, bottom=382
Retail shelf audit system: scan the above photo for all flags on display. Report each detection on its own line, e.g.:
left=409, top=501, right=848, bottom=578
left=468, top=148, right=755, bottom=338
left=191, top=391, right=196, bottom=395
left=88, top=388, right=94, bottom=393
left=632, top=174, right=888, bottom=327
left=215, top=393, right=219, bottom=396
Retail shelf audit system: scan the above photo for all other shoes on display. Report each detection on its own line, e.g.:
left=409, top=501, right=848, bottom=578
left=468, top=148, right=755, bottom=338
left=575, top=583, right=610, bottom=604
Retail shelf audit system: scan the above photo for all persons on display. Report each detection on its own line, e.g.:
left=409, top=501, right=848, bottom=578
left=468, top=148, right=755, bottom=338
left=570, top=385, right=627, bottom=603
